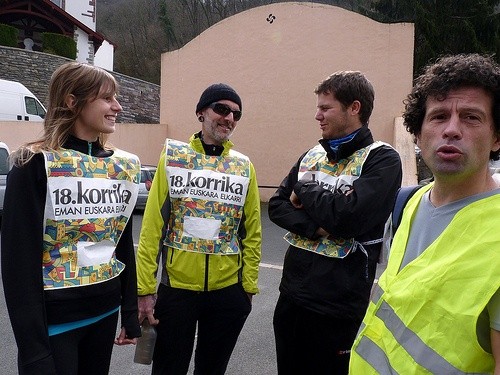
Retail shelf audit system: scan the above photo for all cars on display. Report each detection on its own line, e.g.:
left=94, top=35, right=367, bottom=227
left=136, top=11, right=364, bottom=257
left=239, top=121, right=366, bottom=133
left=134, top=164, right=158, bottom=210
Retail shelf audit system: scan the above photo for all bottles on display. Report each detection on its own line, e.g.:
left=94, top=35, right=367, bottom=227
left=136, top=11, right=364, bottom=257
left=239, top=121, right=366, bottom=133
left=133, top=314, right=158, bottom=365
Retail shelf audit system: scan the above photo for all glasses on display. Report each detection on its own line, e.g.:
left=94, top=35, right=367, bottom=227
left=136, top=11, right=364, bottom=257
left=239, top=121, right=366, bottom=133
left=210, top=103, right=242, bottom=121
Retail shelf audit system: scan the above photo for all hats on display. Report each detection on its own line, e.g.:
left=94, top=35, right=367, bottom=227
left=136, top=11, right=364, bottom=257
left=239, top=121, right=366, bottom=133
left=196, top=83, right=242, bottom=114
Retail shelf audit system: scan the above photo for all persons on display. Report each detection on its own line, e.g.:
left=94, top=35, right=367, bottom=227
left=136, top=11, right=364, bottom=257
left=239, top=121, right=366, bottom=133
left=267, top=72, right=403, bottom=375
left=137, top=84, right=262, bottom=375
left=1, top=62, right=142, bottom=375
left=349, top=53, right=500, bottom=375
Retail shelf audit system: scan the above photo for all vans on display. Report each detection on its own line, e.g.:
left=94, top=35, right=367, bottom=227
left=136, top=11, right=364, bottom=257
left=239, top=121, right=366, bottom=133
left=0, top=142, right=10, bottom=211
left=0, top=79, right=47, bottom=122
